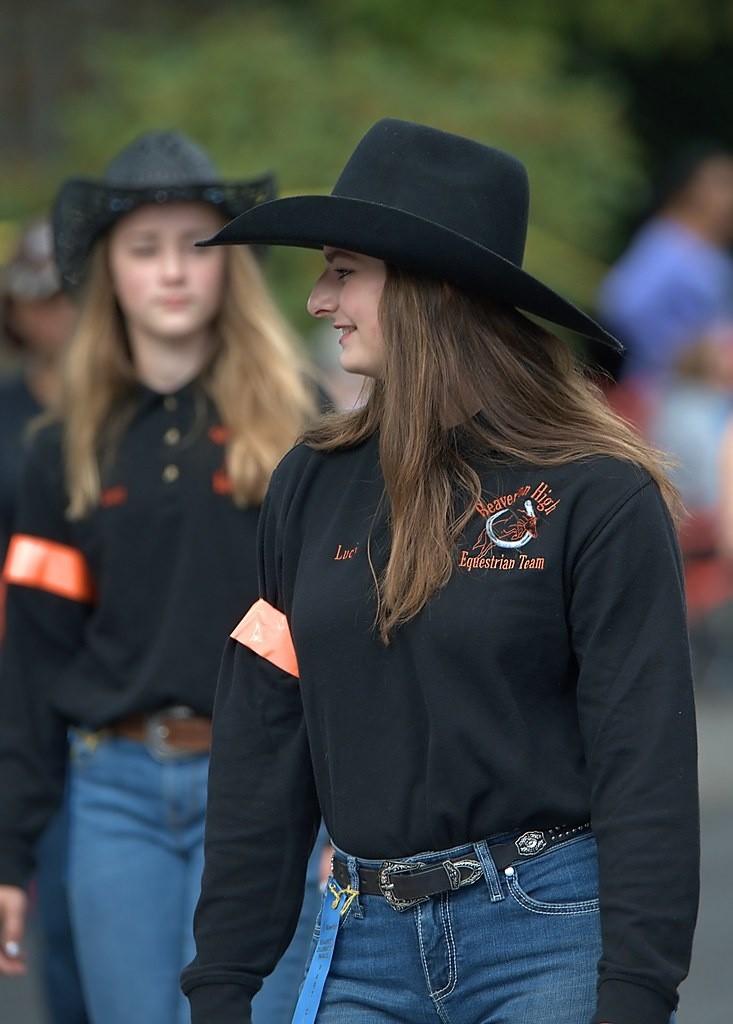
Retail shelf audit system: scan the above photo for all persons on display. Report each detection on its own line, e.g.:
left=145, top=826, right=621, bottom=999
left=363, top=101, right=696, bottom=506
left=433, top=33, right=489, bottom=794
left=595, top=137, right=733, bottom=681
left=1, top=131, right=341, bottom=1024
left=178, top=118, right=701, bottom=1024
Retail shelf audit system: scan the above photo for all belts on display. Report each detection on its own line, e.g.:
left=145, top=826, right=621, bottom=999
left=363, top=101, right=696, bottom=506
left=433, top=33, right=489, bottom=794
left=109, top=706, right=212, bottom=766
left=328, top=815, right=592, bottom=901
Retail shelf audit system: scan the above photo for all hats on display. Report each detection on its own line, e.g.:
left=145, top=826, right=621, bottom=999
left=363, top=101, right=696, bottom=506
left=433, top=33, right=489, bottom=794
left=192, top=116, right=624, bottom=352
left=47, top=129, right=281, bottom=301
